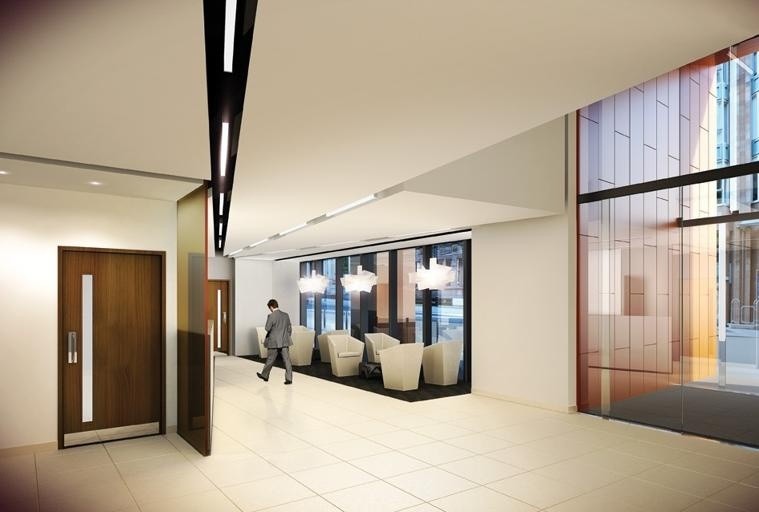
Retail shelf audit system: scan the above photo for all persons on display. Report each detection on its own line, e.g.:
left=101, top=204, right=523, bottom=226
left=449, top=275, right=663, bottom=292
left=256, top=298, right=294, bottom=384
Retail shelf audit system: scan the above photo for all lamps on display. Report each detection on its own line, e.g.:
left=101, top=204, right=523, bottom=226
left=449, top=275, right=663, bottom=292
left=407, top=244, right=456, bottom=291
left=297, top=255, right=378, bottom=295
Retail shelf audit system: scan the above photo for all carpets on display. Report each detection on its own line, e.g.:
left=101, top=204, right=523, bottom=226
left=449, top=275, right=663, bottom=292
left=236, top=347, right=470, bottom=402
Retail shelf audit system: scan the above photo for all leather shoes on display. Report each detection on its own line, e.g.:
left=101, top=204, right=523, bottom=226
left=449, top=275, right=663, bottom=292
left=283, top=380, right=292, bottom=384
left=257, top=372, right=267, bottom=381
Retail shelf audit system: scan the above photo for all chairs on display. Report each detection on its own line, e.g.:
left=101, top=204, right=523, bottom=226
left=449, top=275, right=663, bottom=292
left=255, top=325, right=462, bottom=391
